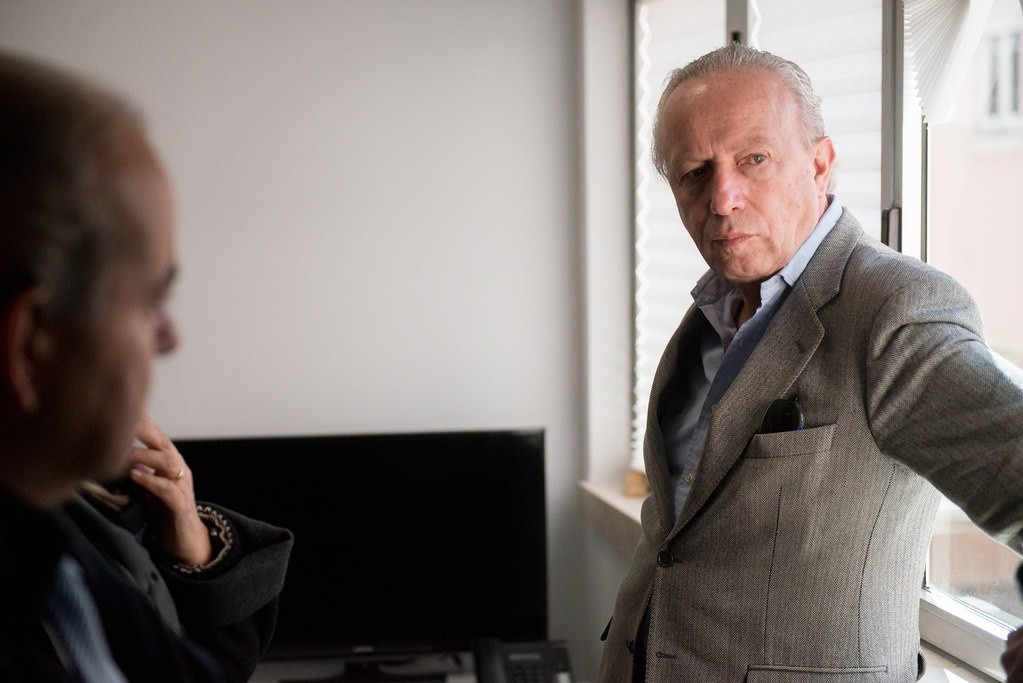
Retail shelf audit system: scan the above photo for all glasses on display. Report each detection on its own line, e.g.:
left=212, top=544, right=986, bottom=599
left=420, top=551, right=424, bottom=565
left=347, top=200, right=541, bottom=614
left=759, top=392, right=805, bottom=434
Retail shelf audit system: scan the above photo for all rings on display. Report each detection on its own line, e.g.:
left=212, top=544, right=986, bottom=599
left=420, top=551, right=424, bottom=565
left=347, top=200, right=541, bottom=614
left=173, top=467, right=184, bottom=479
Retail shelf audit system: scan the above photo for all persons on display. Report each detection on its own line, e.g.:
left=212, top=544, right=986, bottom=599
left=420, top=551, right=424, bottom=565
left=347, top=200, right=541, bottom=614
left=0, top=47, right=299, bottom=683
left=590, top=45, right=1023, bottom=683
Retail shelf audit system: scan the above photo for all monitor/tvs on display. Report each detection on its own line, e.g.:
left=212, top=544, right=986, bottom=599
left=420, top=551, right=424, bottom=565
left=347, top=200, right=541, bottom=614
left=148, top=426, right=550, bottom=683
